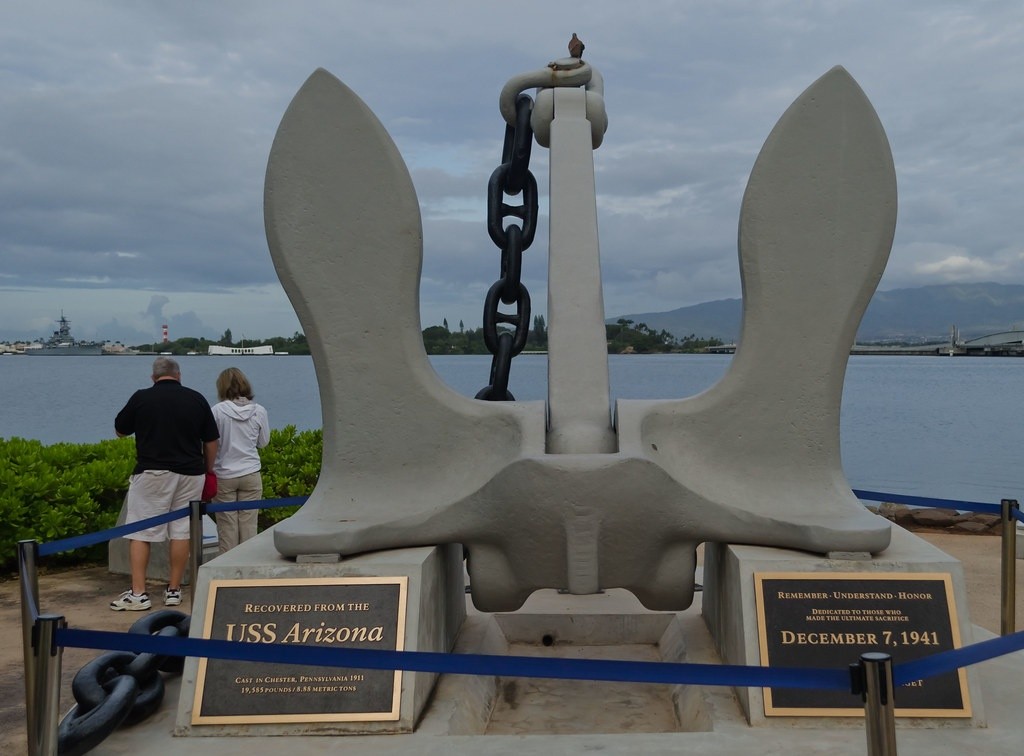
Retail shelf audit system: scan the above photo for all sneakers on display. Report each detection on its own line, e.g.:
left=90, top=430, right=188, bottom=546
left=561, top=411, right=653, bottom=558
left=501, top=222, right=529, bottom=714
left=110, top=589, right=152, bottom=611
left=162, top=584, right=184, bottom=606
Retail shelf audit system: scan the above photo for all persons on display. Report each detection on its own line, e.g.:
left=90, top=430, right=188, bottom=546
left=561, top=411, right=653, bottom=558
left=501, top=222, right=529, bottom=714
left=110, top=358, right=220, bottom=611
left=210, top=368, right=270, bottom=556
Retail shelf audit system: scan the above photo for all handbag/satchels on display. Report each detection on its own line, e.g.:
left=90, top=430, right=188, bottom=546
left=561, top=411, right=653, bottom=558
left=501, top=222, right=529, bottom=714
left=202, top=472, right=218, bottom=500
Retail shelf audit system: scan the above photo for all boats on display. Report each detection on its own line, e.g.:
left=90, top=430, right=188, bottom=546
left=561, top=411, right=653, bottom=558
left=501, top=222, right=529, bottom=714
left=24, top=310, right=104, bottom=356
